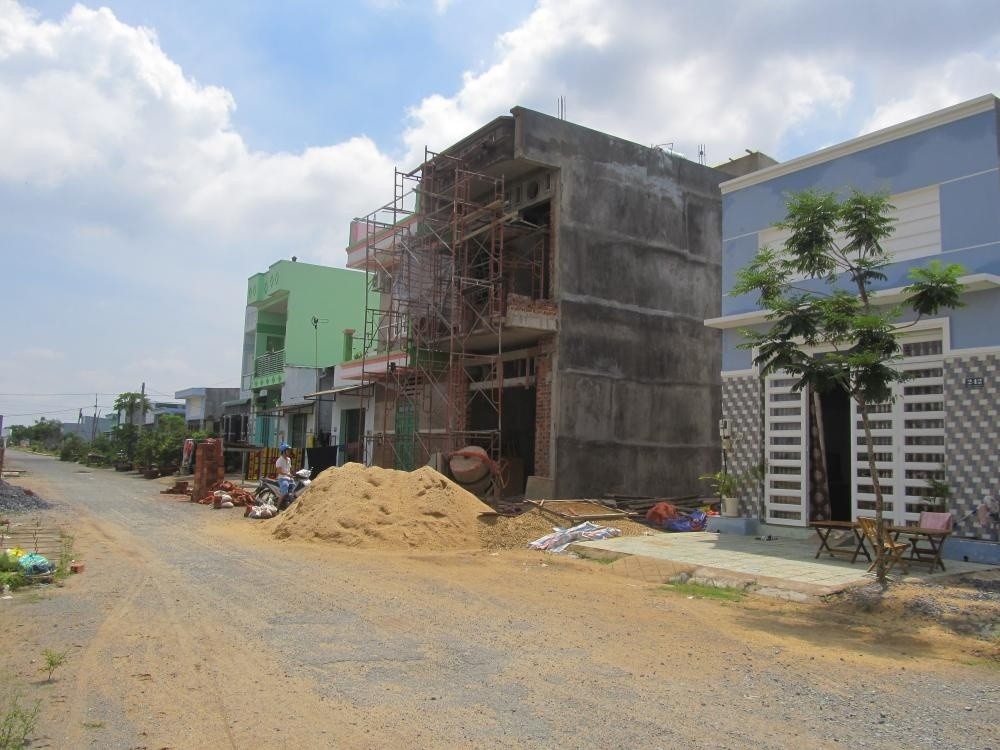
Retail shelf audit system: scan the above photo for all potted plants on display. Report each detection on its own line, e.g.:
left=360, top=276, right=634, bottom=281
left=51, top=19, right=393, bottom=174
left=698, top=465, right=762, bottom=517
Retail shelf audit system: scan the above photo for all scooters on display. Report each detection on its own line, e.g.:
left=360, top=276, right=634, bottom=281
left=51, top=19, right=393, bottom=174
left=252, top=467, right=314, bottom=511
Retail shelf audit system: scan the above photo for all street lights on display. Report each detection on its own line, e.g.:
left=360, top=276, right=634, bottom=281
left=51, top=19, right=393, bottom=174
left=310, top=315, right=330, bottom=449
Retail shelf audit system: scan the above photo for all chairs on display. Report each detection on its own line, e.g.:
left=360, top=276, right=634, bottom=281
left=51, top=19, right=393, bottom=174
left=857, top=516, right=910, bottom=576
left=909, top=511, right=953, bottom=575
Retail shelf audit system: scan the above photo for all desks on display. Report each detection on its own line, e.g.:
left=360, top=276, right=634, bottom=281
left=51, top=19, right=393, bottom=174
left=886, top=525, right=951, bottom=574
left=807, top=521, right=872, bottom=564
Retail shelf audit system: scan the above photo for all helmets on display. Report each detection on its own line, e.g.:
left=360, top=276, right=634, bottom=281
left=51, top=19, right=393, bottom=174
left=280, top=444, right=292, bottom=453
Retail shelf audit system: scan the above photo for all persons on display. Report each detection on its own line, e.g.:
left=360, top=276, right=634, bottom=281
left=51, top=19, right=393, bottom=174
left=276, top=444, right=295, bottom=510
